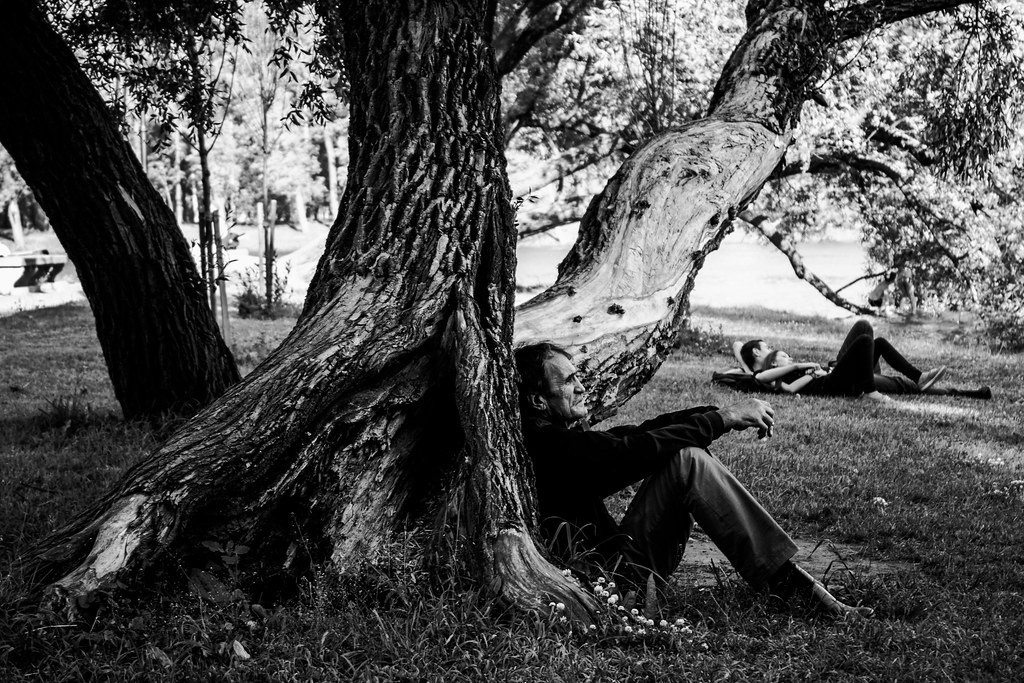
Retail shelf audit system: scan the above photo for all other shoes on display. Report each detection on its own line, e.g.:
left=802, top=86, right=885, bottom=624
left=770, top=563, right=876, bottom=622
left=918, top=364, right=947, bottom=391
left=980, top=386, right=992, bottom=400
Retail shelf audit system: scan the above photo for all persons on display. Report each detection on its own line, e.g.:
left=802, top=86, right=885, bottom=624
left=512, top=343, right=874, bottom=627
left=739, top=319, right=992, bottom=400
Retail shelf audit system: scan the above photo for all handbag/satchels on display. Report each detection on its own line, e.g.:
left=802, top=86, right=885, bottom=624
left=711, top=371, right=785, bottom=395
